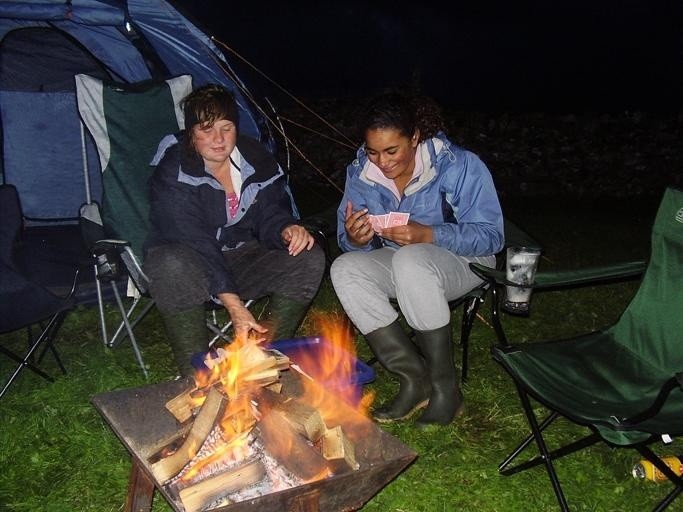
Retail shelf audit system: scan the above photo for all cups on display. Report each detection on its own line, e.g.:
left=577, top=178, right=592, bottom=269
left=505, top=246, right=542, bottom=309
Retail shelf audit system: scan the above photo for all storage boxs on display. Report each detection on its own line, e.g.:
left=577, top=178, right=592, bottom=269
left=192, top=336, right=378, bottom=410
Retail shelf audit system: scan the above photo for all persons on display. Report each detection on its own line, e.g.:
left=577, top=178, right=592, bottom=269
left=142, top=83, right=325, bottom=377
left=330, top=86, right=506, bottom=427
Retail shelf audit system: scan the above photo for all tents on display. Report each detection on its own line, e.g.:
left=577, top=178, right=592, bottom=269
left=1, top=0, right=303, bottom=307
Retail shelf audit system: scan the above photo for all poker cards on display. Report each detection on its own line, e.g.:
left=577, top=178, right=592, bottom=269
left=365, top=212, right=410, bottom=234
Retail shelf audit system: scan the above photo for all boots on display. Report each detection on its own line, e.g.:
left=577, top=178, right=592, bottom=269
left=405, top=316, right=467, bottom=428
left=161, top=306, right=207, bottom=380
left=252, top=291, right=313, bottom=344
left=362, top=317, right=432, bottom=424
